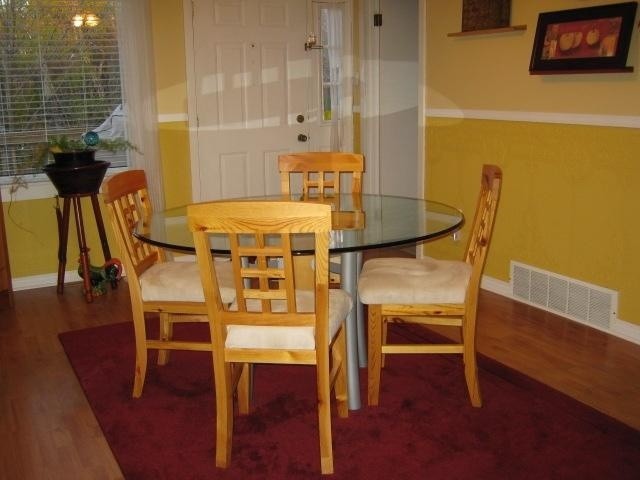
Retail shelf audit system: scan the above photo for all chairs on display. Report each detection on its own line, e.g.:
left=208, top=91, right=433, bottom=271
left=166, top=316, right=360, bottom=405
left=358, top=165, right=503, bottom=408
left=102, top=169, right=251, bottom=398
left=186, top=201, right=335, bottom=474
left=278, top=152, right=366, bottom=288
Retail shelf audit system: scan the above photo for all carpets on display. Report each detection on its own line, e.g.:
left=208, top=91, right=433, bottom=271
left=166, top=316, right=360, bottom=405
left=57, top=316, right=640, bottom=480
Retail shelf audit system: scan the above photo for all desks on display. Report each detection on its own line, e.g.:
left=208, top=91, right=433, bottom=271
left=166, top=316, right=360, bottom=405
left=130, top=193, right=466, bottom=410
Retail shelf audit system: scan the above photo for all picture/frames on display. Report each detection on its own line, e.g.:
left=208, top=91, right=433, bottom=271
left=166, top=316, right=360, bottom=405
left=529, top=2, right=638, bottom=71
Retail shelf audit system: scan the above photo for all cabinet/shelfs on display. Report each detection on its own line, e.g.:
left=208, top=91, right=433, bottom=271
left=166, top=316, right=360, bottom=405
left=445, top=25, right=635, bottom=75
left=53, top=194, right=119, bottom=302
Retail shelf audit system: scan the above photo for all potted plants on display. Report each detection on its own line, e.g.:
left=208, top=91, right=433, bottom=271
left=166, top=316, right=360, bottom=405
left=8, top=134, right=145, bottom=196
